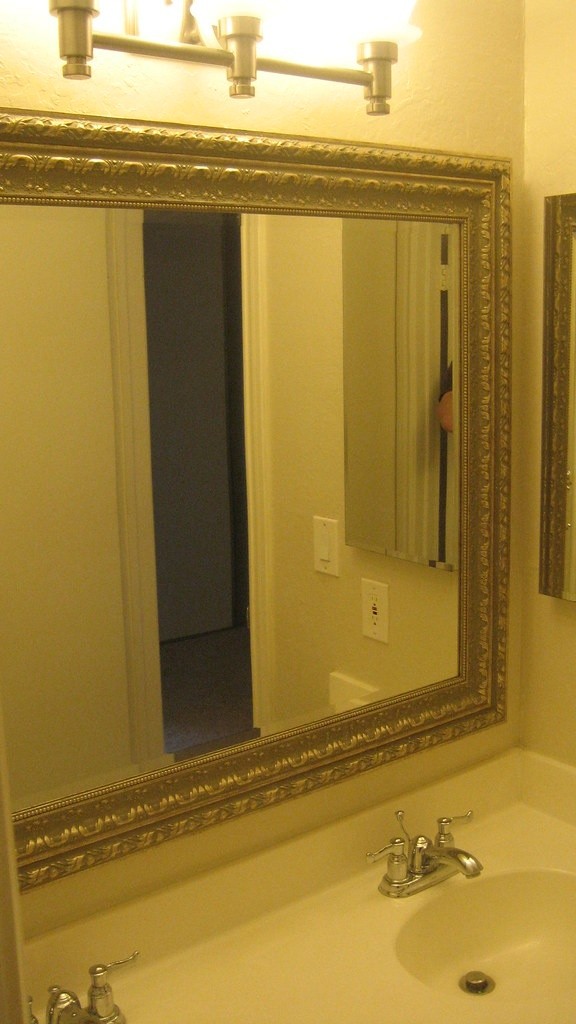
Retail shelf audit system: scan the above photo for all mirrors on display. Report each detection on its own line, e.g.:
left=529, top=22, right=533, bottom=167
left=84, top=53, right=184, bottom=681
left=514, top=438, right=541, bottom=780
left=1, top=106, right=510, bottom=894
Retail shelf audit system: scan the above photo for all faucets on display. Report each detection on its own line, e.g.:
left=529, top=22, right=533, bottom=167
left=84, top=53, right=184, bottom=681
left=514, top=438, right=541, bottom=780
left=44, top=983, right=109, bottom=1024
left=407, top=835, right=484, bottom=880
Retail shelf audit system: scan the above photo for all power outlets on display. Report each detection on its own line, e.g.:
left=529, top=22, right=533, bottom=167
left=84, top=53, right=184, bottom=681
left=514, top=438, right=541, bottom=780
left=356, top=579, right=390, bottom=644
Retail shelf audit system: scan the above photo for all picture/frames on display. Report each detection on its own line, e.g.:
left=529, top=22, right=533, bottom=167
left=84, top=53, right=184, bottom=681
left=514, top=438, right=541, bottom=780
left=538, top=192, right=576, bottom=601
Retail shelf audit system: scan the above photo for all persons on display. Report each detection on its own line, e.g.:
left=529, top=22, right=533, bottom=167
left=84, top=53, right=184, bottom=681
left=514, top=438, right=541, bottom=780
left=439, top=361, right=454, bottom=433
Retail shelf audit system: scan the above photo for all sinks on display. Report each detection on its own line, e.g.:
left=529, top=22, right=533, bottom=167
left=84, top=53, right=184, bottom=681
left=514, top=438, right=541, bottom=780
left=391, top=864, right=576, bottom=1010
